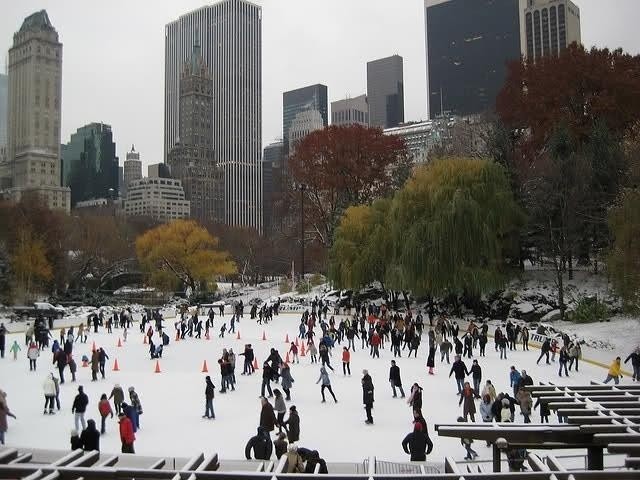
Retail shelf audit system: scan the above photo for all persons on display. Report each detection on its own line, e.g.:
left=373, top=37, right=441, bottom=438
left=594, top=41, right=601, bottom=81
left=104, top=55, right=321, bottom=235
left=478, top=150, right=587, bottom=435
left=1, top=290, right=639, bottom=475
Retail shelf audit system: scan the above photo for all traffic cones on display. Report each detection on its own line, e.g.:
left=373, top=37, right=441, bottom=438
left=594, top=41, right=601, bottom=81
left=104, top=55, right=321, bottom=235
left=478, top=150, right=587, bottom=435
left=253, top=330, right=305, bottom=370
left=83, top=360, right=88, bottom=367
left=92, top=341, right=96, bottom=351
left=113, top=359, right=120, bottom=370
left=155, top=360, right=160, bottom=372
left=175, top=328, right=241, bottom=341
left=117, top=338, right=123, bottom=347
left=143, top=335, right=148, bottom=344
left=202, top=360, right=210, bottom=372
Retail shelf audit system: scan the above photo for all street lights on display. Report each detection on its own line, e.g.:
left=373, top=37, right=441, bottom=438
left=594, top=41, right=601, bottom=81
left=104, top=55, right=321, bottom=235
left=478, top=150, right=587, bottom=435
left=297, top=182, right=310, bottom=280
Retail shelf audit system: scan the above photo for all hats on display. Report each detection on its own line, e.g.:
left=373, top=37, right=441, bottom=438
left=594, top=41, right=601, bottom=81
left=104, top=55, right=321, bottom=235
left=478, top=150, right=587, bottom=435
left=416, top=422, right=422, bottom=430
left=290, top=406, right=296, bottom=411
